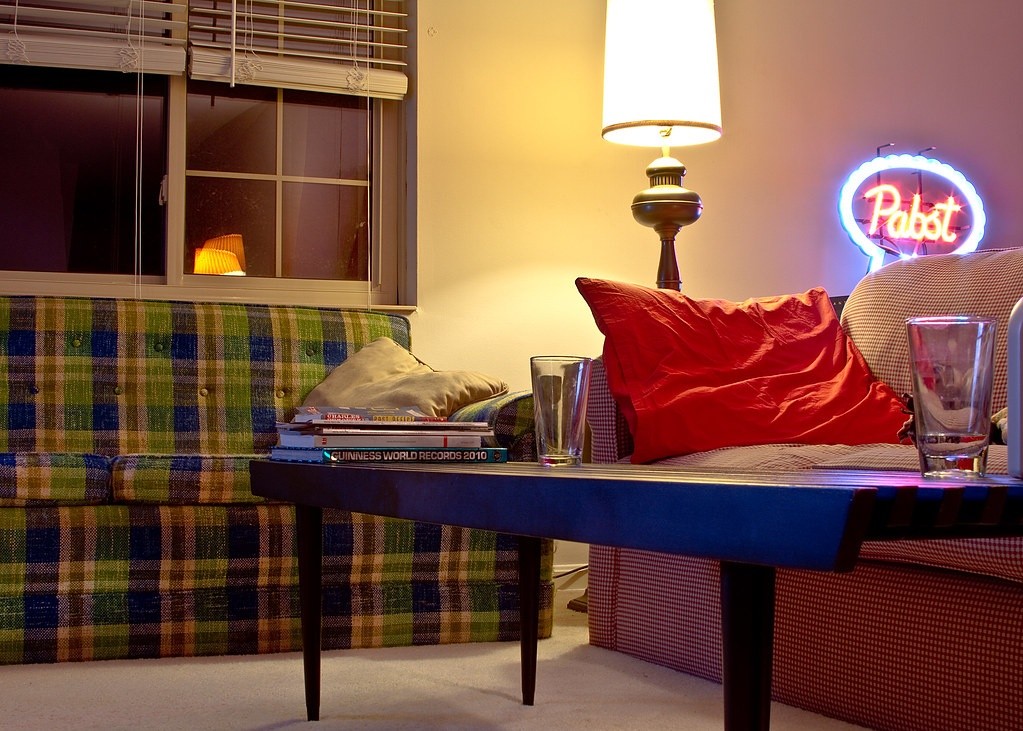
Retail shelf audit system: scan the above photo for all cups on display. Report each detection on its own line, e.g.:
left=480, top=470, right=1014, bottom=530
left=530, top=356, right=590, bottom=468
left=903, top=316, right=1000, bottom=482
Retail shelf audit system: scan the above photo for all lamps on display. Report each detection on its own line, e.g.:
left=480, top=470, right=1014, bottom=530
left=191, top=232, right=249, bottom=276
left=600, top=0, right=723, bottom=290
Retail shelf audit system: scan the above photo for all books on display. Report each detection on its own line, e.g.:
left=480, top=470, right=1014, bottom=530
left=268, top=405, right=509, bottom=464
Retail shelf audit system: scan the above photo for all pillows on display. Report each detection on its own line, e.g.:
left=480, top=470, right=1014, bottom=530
left=276, top=334, right=512, bottom=449
left=574, top=278, right=919, bottom=466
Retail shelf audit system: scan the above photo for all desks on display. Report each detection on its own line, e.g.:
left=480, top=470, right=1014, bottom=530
left=236, top=448, right=1022, bottom=731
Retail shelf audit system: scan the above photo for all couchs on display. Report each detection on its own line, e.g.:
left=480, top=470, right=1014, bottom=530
left=0, top=295, right=559, bottom=665
left=583, top=232, right=1023, bottom=731
